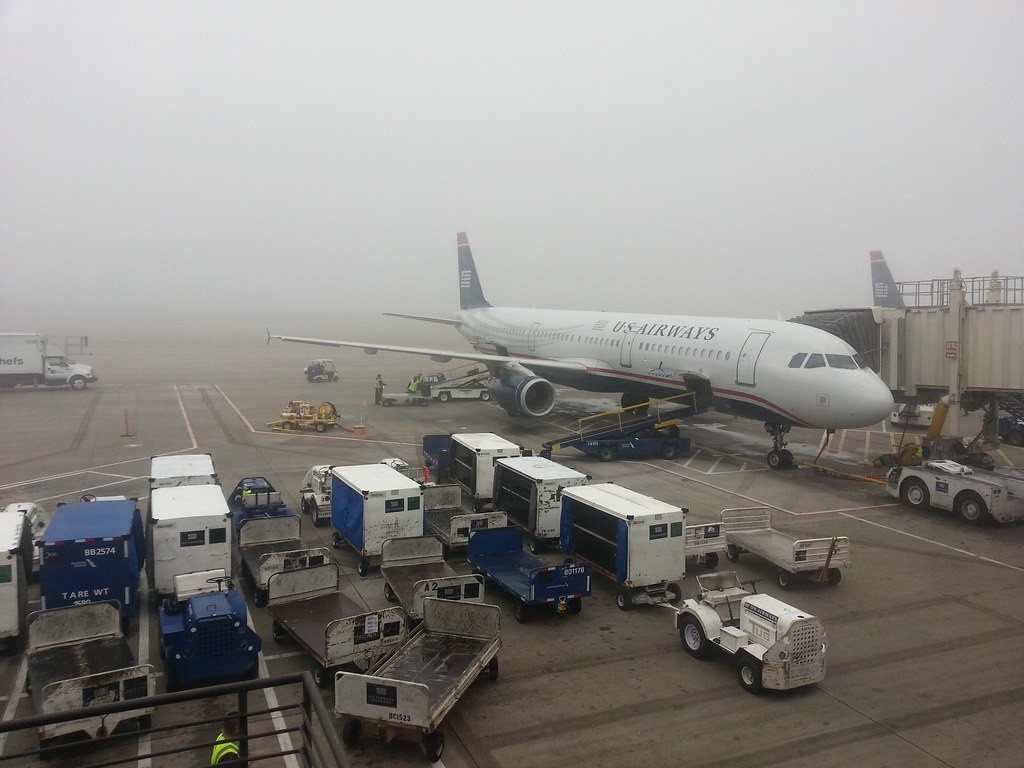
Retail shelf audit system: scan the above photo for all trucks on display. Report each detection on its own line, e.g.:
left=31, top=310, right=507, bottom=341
left=142, top=451, right=262, bottom=700
left=300, top=464, right=503, bottom=764
left=0, top=333, right=98, bottom=390
left=227, top=476, right=408, bottom=688
left=423, top=430, right=827, bottom=697
left=0, top=504, right=47, bottom=645
left=21, top=496, right=153, bottom=758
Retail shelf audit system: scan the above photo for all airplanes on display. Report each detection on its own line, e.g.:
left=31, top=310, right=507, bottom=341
left=269, top=229, right=896, bottom=471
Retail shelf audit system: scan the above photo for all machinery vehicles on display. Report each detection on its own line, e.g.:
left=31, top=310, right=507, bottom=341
left=281, top=400, right=342, bottom=432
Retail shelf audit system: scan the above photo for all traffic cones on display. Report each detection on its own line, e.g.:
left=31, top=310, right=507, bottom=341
left=120, top=409, right=134, bottom=436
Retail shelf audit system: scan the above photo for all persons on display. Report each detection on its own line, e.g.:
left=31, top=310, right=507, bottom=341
left=212, top=711, right=241, bottom=767
left=404, top=373, right=424, bottom=403
left=374, top=374, right=386, bottom=404
